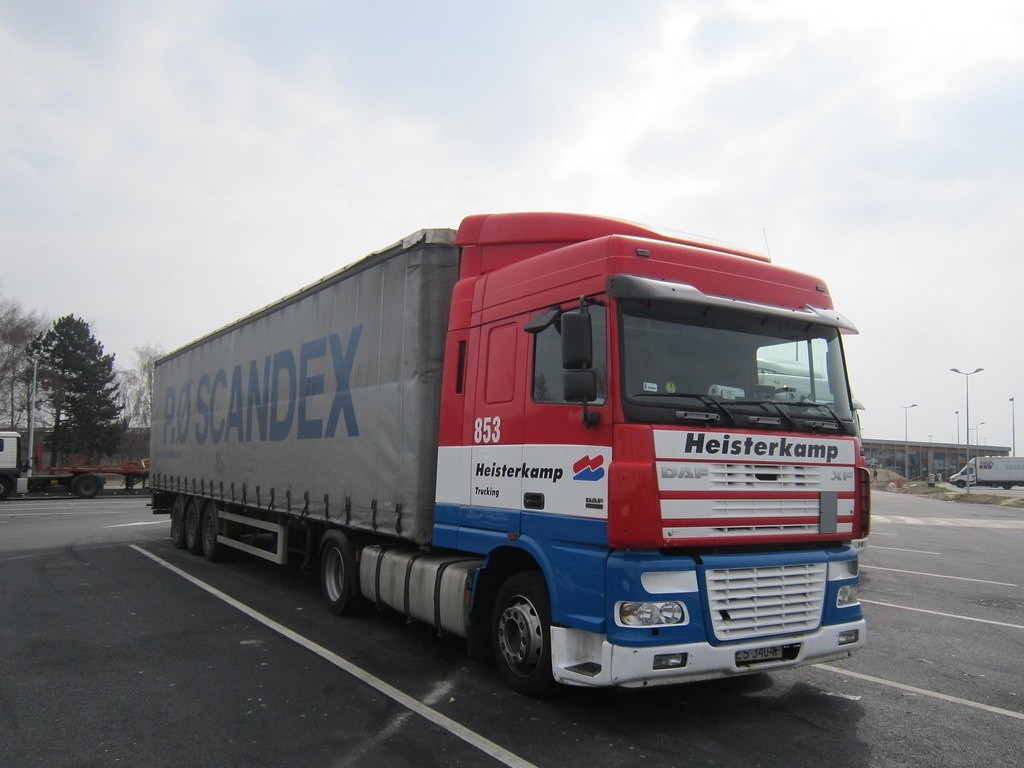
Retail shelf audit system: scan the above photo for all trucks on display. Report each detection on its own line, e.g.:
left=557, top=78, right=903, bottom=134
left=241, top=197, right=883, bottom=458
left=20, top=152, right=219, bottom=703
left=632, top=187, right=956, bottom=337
left=949, top=455, right=1024, bottom=490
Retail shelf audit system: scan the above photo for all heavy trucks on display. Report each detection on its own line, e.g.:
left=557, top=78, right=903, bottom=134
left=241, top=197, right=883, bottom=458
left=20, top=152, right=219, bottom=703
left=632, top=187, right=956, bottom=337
left=144, top=209, right=872, bottom=705
left=0, top=431, right=149, bottom=499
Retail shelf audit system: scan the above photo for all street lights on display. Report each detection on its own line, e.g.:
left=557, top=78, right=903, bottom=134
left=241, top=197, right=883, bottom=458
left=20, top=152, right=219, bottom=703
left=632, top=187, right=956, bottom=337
left=1010, top=398, right=1016, bottom=457
left=954, top=411, right=961, bottom=472
left=975, top=421, right=985, bottom=486
left=29, top=353, right=40, bottom=476
left=901, top=403, right=918, bottom=484
left=950, top=367, right=984, bottom=494
